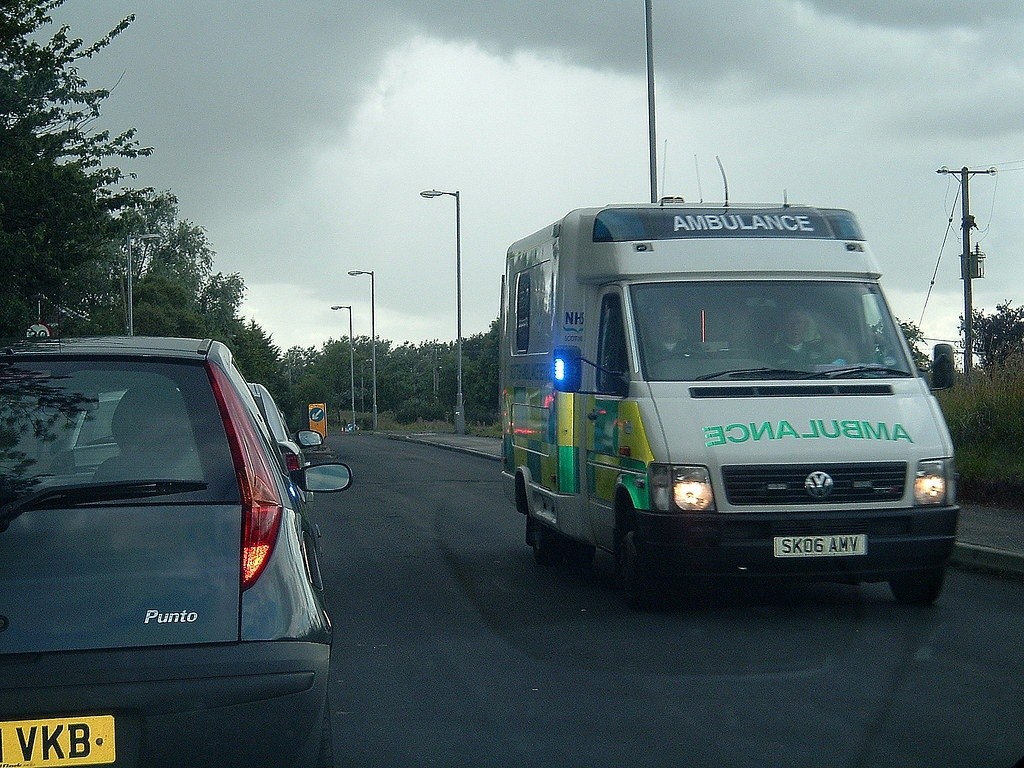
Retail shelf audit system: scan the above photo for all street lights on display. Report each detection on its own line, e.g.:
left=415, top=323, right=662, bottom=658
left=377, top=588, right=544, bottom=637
left=347, top=269, right=377, bottom=431
left=420, top=189, right=465, bottom=435
left=126, top=232, right=161, bottom=336
left=330, top=305, right=356, bottom=429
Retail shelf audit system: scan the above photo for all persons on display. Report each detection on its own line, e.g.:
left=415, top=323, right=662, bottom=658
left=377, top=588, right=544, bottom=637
left=646, top=306, right=704, bottom=365
left=763, top=307, right=856, bottom=369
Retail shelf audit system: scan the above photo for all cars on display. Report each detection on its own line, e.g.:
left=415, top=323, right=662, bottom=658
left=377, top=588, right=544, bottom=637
left=68, top=384, right=324, bottom=508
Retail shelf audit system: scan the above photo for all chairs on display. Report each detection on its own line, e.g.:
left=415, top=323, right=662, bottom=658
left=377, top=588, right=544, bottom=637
left=93, top=382, right=205, bottom=482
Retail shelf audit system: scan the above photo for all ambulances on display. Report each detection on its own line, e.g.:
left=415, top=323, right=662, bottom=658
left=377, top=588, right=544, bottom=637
left=498, top=198, right=959, bottom=611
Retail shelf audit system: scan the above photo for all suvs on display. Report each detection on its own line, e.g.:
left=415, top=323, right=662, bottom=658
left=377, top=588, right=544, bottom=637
left=0, top=336, right=354, bottom=768
